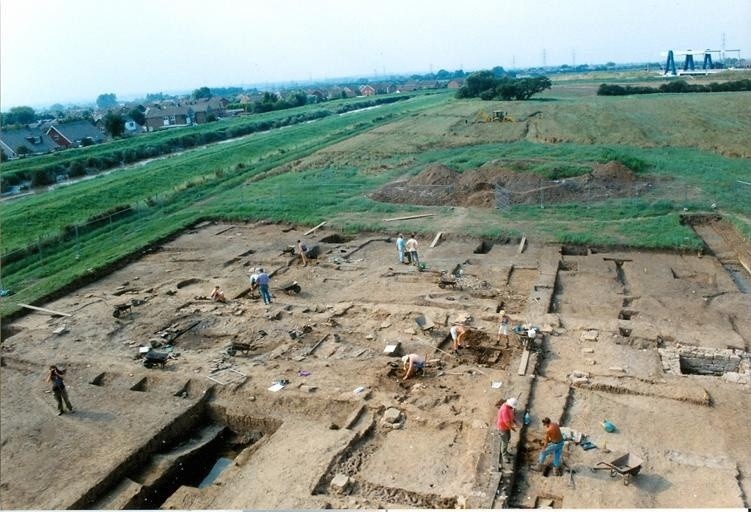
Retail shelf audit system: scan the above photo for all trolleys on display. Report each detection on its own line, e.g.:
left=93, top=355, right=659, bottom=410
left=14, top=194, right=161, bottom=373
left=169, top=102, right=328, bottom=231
left=596, top=452, right=643, bottom=486
left=515, top=329, right=539, bottom=351
left=112, top=298, right=137, bottom=318
left=145, top=352, right=169, bottom=368
left=437, top=280, right=456, bottom=288
left=281, top=246, right=294, bottom=257
left=227, top=343, right=264, bottom=356
left=270, top=280, right=300, bottom=296
left=415, top=314, right=435, bottom=335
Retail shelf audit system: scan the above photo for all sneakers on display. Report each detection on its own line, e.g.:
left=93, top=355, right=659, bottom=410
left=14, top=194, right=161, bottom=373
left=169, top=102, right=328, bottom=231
left=57, top=408, right=75, bottom=416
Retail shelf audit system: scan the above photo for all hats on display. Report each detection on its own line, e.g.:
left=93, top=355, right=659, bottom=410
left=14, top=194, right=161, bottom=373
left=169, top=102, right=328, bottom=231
left=402, top=354, right=409, bottom=364
left=506, top=397, right=518, bottom=409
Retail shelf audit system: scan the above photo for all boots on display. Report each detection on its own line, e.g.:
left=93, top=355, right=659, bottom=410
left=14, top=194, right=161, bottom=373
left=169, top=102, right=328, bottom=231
left=530, top=463, right=542, bottom=472
left=552, top=466, right=558, bottom=472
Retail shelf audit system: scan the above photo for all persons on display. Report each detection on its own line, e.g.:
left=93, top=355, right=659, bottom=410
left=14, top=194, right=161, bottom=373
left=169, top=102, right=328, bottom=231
left=256, top=268, right=273, bottom=305
left=406, top=234, right=421, bottom=267
left=396, top=233, right=406, bottom=263
left=492, top=309, right=514, bottom=349
left=402, top=352, right=425, bottom=381
left=211, top=285, right=226, bottom=303
left=496, top=397, right=523, bottom=464
left=46, top=365, right=75, bottom=417
left=450, top=326, right=472, bottom=353
left=529, top=417, right=565, bottom=477
left=296, top=239, right=308, bottom=267
left=249, top=273, right=272, bottom=297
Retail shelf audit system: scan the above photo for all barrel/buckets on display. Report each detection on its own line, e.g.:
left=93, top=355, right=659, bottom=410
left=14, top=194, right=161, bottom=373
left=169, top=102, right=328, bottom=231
left=419, top=262, right=426, bottom=270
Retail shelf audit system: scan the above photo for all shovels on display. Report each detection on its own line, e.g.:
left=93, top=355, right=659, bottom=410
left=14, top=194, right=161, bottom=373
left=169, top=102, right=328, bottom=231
left=292, top=334, right=328, bottom=362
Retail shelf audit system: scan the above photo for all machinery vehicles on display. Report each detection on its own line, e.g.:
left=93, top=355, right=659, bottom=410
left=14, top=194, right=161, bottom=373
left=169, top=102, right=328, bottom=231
left=471, top=109, right=516, bottom=124
